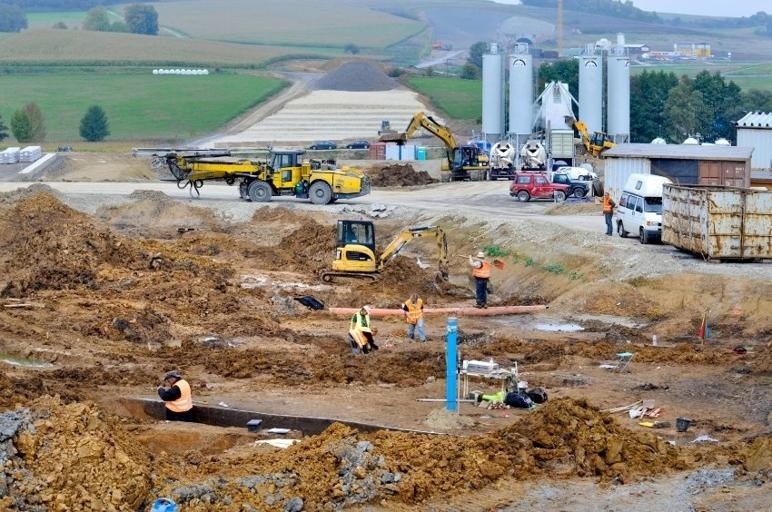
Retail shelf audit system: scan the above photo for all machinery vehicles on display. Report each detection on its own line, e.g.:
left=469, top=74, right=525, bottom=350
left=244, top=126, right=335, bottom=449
left=318, top=218, right=449, bottom=287
left=130, top=145, right=371, bottom=205
left=486, top=140, right=517, bottom=180
left=378, top=112, right=487, bottom=182
left=520, top=138, right=546, bottom=170
left=570, top=115, right=617, bottom=160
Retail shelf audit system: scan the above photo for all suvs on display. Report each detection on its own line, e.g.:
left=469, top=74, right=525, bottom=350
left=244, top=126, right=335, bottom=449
left=507, top=159, right=595, bottom=201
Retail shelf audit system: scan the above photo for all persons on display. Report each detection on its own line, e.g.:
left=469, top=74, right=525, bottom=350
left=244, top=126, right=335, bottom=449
left=468, top=251, right=492, bottom=309
left=346, top=326, right=381, bottom=357
left=598, top=191, right=616, bottom=236
left=402, top=292, right=427, bottom=342
left=156, top=370, right=194, bottom=423
left=350, top=304, right=372, bottom=351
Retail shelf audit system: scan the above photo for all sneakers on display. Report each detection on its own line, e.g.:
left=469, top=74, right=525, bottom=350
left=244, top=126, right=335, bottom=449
left=473, top=303, right=488, bottom=308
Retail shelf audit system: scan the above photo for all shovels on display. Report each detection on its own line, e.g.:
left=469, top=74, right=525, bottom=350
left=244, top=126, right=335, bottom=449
left=457, top=253, right=505, bottom=271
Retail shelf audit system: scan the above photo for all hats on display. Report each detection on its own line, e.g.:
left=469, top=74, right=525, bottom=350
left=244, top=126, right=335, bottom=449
left=164, top=370, right=182, bottom=381
left=363, top=305, right=371, bottom=314
left=477, top=252, right=485, bottom=258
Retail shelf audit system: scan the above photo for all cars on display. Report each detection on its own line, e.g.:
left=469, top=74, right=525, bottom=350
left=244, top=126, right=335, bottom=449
left=310, top=140, right=337, bottom=149
left=346, top=140, right=370, bottom=149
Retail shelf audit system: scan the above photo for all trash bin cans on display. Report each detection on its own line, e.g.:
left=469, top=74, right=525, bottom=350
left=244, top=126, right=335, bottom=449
left=417, top=148, right=425, bottom=161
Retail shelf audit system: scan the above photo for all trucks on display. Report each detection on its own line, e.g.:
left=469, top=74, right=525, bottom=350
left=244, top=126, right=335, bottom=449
left=615, top=172, right=675, bottom=245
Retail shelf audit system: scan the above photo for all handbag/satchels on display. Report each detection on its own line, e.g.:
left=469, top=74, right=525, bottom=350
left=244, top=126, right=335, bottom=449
left=506, top=385, right=547, bottom=408
